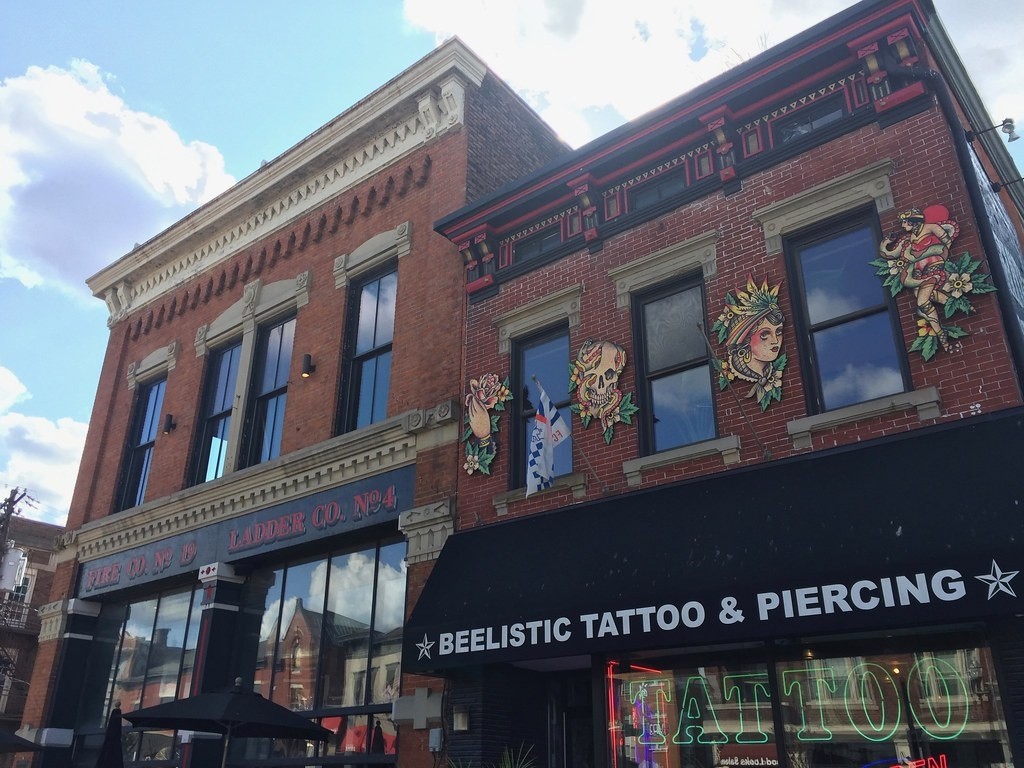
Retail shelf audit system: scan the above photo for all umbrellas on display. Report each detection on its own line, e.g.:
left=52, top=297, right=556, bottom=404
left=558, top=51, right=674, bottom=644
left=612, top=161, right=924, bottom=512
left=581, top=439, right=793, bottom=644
left=0, top=729, right=47, bottom=753
left=121, top=677, right=335, bottom=768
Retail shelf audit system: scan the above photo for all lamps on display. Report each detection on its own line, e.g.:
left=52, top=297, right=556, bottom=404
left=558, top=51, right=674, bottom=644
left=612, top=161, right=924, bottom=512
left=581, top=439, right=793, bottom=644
left=965, top=118, right=1021, bottom=143
left=301, top=354, right=316, bottom=378
left=162, top=413, right=176, bottom=434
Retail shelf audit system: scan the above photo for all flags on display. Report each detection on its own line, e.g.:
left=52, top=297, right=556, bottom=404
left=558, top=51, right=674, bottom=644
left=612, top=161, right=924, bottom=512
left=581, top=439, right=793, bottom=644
left=524, top=391, right=571, bottom=500
